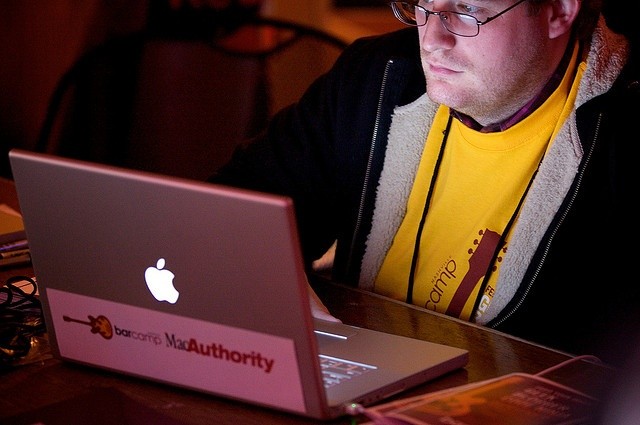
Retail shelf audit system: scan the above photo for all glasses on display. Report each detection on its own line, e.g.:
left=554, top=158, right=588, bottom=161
left=390, top=1, right=526, bottom=38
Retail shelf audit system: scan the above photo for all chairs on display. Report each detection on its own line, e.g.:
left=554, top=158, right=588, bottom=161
left=0, top=177, right=639, bottom=424
left=32, top=15, right=352, bottom=181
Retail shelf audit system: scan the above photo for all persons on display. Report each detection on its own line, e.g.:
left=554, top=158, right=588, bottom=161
left=200, top=0, right=640, bottom=362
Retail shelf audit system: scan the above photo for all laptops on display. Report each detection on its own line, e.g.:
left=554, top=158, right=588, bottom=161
left=8, top=150, right=470, bottom=420
